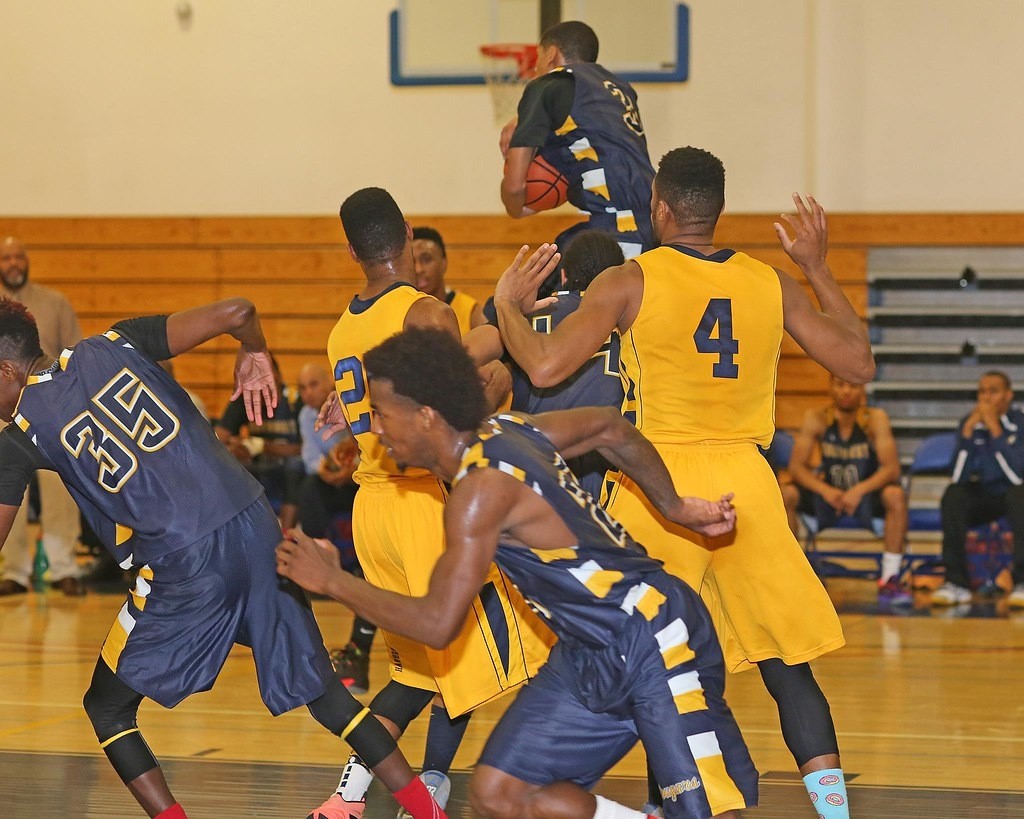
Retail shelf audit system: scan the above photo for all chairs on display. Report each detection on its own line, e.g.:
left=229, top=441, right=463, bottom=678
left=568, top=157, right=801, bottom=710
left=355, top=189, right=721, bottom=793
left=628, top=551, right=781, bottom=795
left=755, top=431, right=793, bottom=478
left=802, top=515, right=893, bottom=583
left=898, top=431, right=1009, bottom=604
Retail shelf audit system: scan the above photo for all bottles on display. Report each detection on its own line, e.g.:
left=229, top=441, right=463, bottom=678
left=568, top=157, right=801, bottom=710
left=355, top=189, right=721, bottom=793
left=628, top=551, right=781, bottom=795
left=31, top=532, right=52, bottom=594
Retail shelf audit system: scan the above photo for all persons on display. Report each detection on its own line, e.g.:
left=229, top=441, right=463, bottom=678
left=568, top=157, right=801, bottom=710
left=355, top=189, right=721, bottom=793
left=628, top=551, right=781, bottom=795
left=929, top=371, right=1024, bottom=607
left=215, top=344, right=299, bottom=517
left=0, top=297, right=451, bottom=819
left=498, top=20, right=657, bottom=300
left=0, top=236, right=87, bottom=596
left=789, top=373, right=914, bottom=606
left=276, top=187, right=879, bottom=818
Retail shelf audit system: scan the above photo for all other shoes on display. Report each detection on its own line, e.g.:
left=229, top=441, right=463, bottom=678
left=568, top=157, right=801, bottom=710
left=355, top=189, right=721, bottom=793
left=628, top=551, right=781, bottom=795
left=63, top=578, right=87, bottom=596
left=329, top=642, right=368, bottom=693
left=1007, top=584, right=1024, bottom=607
left=878, top=574, right=915, bottom=606
left=930, top=581, right=972, bottom=604
left=0, top=580, right=27, bottom=596
left=306, top=794, right=366, bottom=819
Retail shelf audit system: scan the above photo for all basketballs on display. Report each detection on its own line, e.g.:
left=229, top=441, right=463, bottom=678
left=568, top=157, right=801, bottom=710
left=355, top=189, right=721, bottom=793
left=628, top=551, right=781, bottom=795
left=503, top=150, right=571, bottom=212
